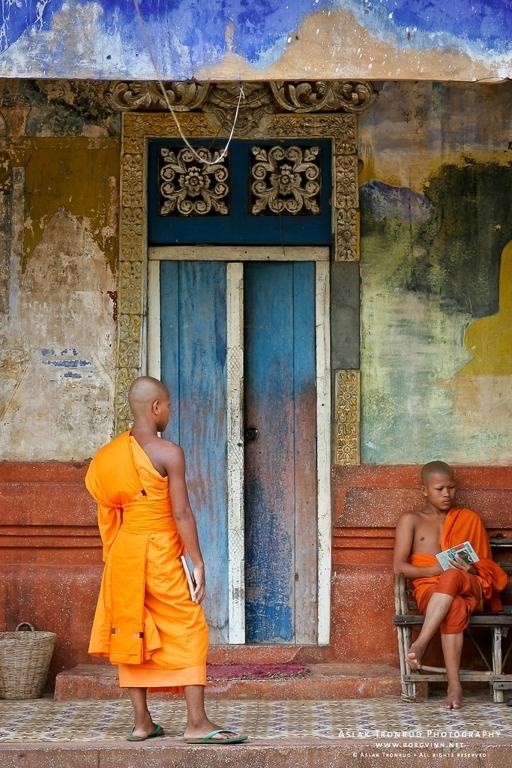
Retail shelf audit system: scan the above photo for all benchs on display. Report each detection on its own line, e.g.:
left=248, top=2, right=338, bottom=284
left=391, top=537, right=512, bottom=703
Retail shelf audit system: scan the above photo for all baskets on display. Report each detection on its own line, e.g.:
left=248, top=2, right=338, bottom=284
left=0, top=622, right=57, bottom=700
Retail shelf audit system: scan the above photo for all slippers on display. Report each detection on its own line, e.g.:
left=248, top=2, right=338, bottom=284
left=127, top=724, right=165, bottom=741
left=184, top=730, right=248, bottom=744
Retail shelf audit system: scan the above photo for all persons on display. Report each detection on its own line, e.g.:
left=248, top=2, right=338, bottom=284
left=84, top=375, right=250, bottom=745
left=392, top=460, right=508, bottom=711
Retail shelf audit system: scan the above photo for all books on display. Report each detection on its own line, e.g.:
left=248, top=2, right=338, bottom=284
left=180, top=551, right=200, bottom=603
left=434, top=541, right=481, bottom=571
